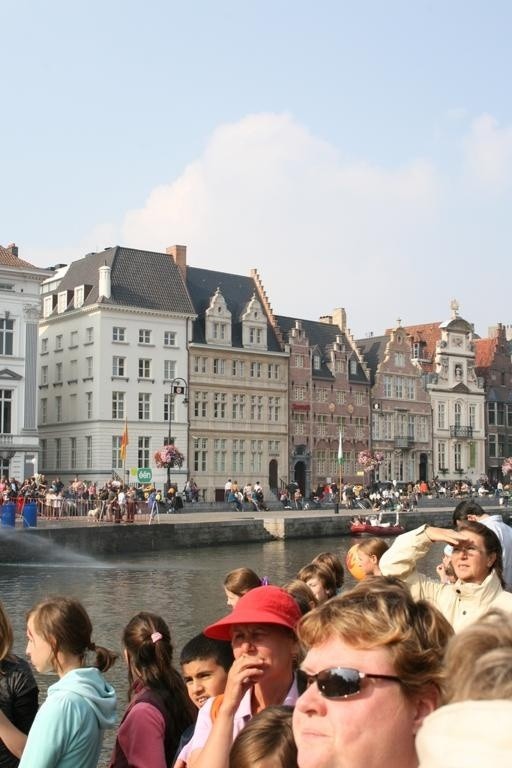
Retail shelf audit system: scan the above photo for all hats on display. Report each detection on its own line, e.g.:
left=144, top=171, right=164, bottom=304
left=203, top=585, right=302, bottom=642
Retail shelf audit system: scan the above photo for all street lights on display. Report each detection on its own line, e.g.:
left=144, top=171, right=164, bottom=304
left=369, top=396, right=384, bottom=484
left=167, top=377, right=187, bottom=488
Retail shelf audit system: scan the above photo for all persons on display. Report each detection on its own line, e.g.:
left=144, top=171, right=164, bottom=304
left=225, top=478, right=512, bottom=513
left=2, top=501, right=511, bottom=767
left=1, top=474, right=201, bottom=525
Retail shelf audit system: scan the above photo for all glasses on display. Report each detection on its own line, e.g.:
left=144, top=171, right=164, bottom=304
left=295, top=667, right=403, bottom=699
left=450, top=544, right=481, bottom=554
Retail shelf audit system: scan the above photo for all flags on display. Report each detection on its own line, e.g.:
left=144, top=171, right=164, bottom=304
left=121, top=421, right=128, bottom=462
